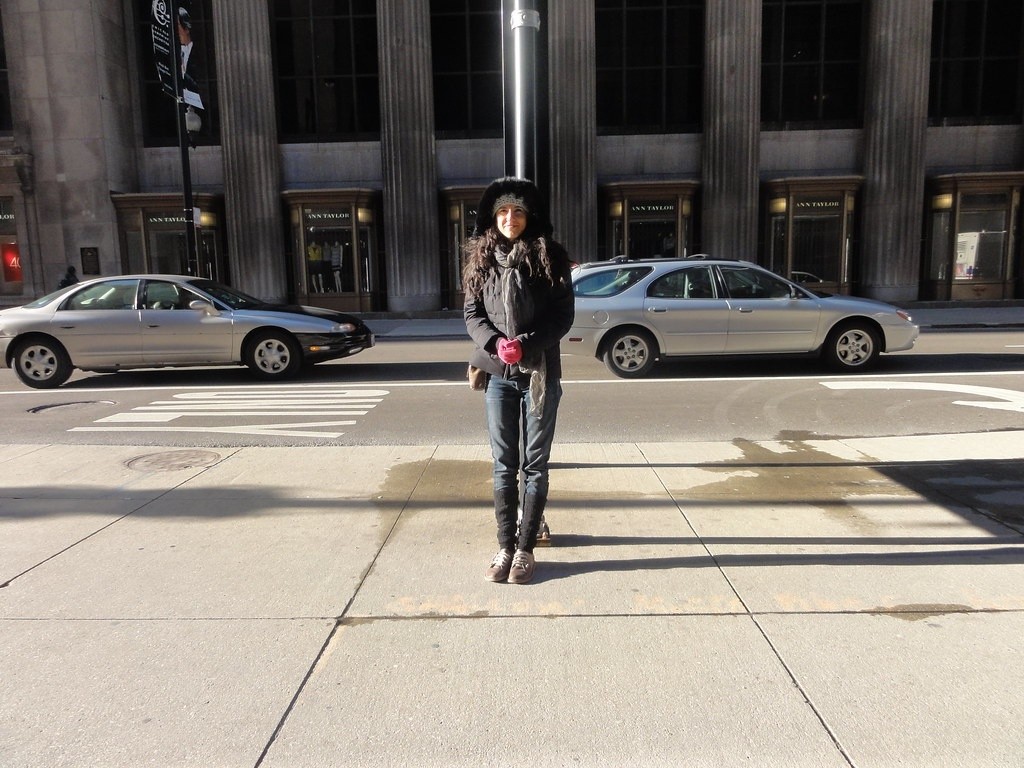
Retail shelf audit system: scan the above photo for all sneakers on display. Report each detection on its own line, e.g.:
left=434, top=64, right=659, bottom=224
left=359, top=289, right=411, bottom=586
left=484, top=548, right=515, bottom=581
left=506, top=548, right=536, bottom=583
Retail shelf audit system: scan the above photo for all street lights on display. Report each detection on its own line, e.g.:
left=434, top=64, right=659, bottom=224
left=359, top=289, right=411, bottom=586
left=176, top=104, right=201, bottom=275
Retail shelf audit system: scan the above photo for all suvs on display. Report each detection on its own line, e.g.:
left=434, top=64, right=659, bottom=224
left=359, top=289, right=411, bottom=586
left=558, top=256, right=920, bottom=380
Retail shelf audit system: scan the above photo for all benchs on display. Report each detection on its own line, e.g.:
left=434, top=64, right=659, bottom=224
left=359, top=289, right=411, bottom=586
left=79, top=298, right=124, bottom=310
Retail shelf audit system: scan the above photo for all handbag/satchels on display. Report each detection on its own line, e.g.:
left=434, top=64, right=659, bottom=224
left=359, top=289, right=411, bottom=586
left=466, top=361, right=487, bottom=393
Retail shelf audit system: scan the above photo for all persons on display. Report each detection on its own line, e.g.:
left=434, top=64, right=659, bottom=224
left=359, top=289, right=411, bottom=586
left=177, top=6, right=199, bottom=94
left=58, top=265, right=80, bottom=290
left=306, top=240, right=343, bottom=294
left=461, top=176, right=574, bottom=584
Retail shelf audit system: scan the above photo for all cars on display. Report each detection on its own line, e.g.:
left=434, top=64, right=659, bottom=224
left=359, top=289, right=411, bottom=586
left=0, top=275, right=375, bottom=389
left=791, top=271, right=840, bottom=294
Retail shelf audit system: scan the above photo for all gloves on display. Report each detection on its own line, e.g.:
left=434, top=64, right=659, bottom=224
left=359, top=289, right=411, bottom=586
left=496, top=337, right=523, bottom=365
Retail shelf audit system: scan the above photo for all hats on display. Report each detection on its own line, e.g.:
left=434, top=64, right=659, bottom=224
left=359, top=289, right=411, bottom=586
left=492, top=193, right=529, bottom=217
